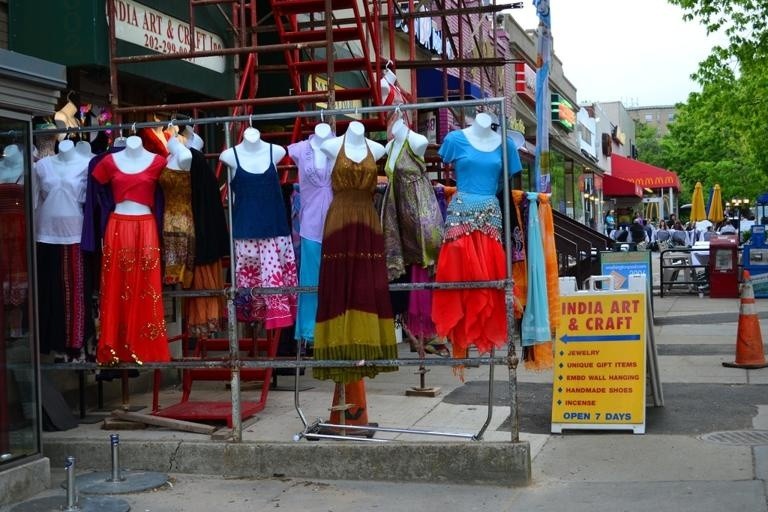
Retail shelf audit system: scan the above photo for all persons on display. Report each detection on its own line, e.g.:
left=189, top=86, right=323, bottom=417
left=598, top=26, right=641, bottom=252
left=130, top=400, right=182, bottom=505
left=287, top=122, right=341, bottom=345
left=218, top=128, right=302, bottom=330
left=1, top=101, right=230, bottom=381
left=380, top=118, right=444, bottom=280
left=378, top=69, right=412, bottom=143
left=433, top=110, right=521, bottom=355
left=315, top=118, right=398, bottom=384
left=603, top=210, right=767, bottom=252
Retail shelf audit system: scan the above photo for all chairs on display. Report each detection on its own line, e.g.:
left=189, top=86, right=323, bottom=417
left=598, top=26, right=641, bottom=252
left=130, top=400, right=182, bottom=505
left=606, top=228, right=742, bottom=247
left=655, top=238, right=693, bottom=296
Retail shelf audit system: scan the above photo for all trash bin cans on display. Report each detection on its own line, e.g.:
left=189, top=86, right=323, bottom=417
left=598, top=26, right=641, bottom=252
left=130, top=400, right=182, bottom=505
left=709, top=235, right=738, bottom=298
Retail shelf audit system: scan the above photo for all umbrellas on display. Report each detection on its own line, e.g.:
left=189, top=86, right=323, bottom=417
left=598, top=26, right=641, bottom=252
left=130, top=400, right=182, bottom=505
left=707, top=184, right=726, bottom=227
left=690, top=182, right=708, bottom=227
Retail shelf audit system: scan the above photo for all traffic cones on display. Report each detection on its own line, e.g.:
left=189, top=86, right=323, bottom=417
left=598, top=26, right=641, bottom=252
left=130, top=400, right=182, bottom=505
left=722, top=268, right=768, bottom=369
left=320, top=374, right=380, bottom=439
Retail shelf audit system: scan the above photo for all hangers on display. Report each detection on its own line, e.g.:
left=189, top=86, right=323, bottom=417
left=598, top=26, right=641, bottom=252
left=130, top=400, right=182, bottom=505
left=1, top=86, right=510, bottom=152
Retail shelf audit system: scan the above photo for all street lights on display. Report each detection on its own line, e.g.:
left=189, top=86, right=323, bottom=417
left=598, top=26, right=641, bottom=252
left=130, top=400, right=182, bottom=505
left=725, top=197, right=750, bottom=246
